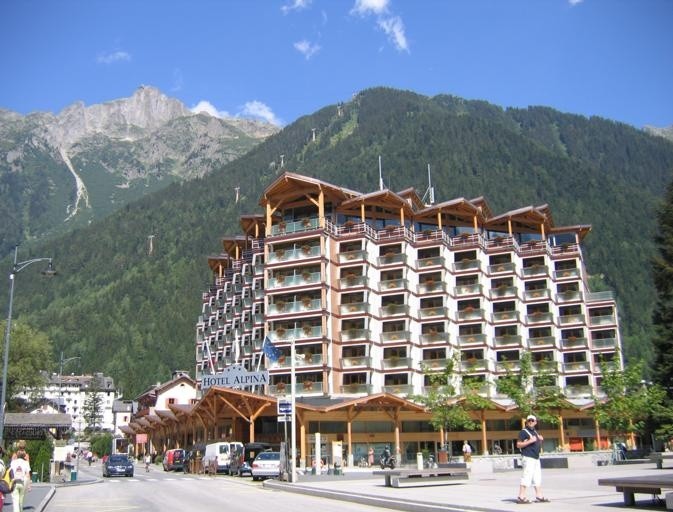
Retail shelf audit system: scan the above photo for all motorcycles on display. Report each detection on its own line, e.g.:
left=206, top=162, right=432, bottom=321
left=377, top=454, right=395, bottom=469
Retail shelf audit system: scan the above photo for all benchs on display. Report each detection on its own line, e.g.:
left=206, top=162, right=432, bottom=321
left=373, top=467, right=471, bottom=487
left=598, top=475, right=673, bottom=505
left=514, top=458, right=569, bottom=468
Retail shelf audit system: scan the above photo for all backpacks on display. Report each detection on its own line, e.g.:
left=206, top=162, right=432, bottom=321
left=0, top=463, right=16, bottom=495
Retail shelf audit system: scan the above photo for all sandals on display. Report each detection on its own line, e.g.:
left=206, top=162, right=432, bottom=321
left=515, top=496, right=532, bottom=504
left=533, top=495, right=551, bottom=502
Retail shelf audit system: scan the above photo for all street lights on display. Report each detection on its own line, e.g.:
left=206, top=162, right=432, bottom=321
left=58, top=351, right=82, bottom=395
left=638, top=379, right=653, bottom=394
left=0, top=244, right=58, bottom=453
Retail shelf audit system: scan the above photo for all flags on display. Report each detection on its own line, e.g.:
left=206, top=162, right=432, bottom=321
left=261, top=336, right=281, bottom=361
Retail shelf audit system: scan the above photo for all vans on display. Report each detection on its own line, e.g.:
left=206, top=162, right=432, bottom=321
left=158, top=440, right=288, bottom=482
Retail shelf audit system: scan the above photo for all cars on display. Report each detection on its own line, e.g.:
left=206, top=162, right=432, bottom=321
left=102, top=453, right=133, bottom=477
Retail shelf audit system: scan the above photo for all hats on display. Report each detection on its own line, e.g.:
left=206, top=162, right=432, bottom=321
left=526, top=414, right=536, bottom=420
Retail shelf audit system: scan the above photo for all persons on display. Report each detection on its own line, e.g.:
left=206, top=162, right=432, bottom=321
left=380, top=444, right=391, bottom=467
left=143, top=452, right=151, bottom=472
left=0, top=446, right=7, bottom=511
left=462, top=439, right=472, bottom=462
left=516, top=414, right=550, bottom=503
left=11, top=438, right=30, bottom=490
left=427, top=454, right=438, bottom=477
left=367, top=446, right=374, bottom=465
left=9, top=448, right=32, bottom=511
left=86, top=450, right=92, bottom=465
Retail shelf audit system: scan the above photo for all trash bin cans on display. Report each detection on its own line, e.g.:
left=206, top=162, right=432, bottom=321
left=416, top=451, right=423, bottom=470
left=438, top=451, right=447, bottom=463
left=32, top=472, right=39, bottom=483
left=71, top=471, right=77, bottom=480
left=656, top=457, right=663, bottom=469
